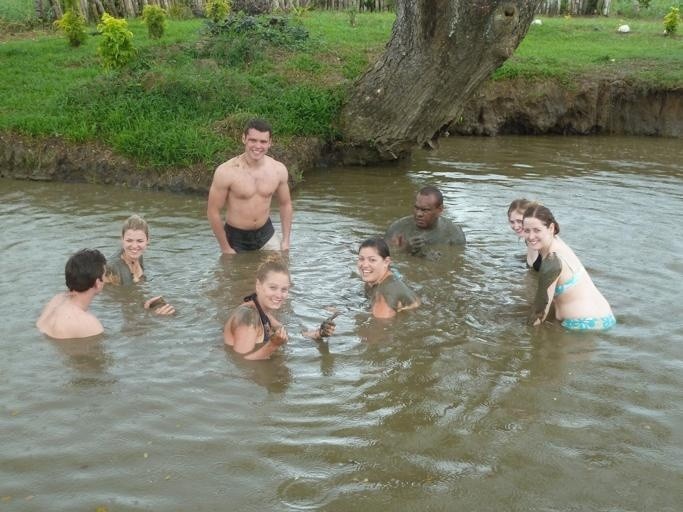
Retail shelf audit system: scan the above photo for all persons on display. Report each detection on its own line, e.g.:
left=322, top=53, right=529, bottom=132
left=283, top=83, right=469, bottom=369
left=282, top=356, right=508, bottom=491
left=206, top=116, right=294, bottom=256
left=223, top=251, right=337, bottom=361
left=381, top=185, right=467, bottom=258
left=356, top=235, right=423, bottom=321
left=520, top=202, right=618, bottom=331
left=506, top=198, right=543, bottom=272
left=103, top=212, right=151, bottom=289
left=34, top=246, right=176, bottom=342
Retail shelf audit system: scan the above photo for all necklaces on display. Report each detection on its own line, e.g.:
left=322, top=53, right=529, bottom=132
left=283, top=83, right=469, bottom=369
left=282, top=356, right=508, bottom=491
left=126, top=258, right=138, bottom=266
